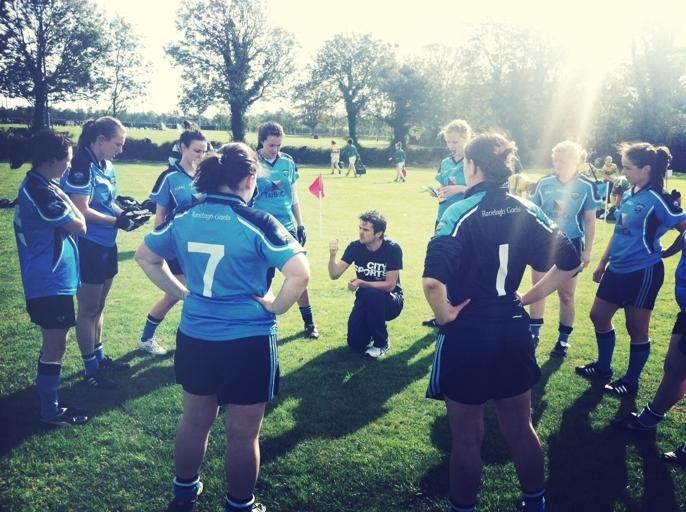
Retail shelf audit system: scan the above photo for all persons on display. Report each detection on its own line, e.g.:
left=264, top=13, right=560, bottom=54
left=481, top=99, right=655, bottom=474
left=246, top=120, right=321, bottom=342
left=329, top=139, right=342, bottom=176
left=115, top=128, right=209, bottom=359
left=169, top=117, right=215, bottom=169
left=421, top=118, right=475, bottom=330
left=608, top=226, right=686, bottom=468
left=508, top=152, right=683, bottom=222
left=387, top=140, right=406, bottom=183
left=526, top=139, right=599, bottom=359
left=59, top=115, right=153, bottom=392
left=343, top=137, right=360, bottom=178
left=422, top=131, right=583, bottom=512
left=328, top=210, right=405, bottom=359
left=135, top=141, right=310, bottom=512
left=573, top=139, right=685, bottom=401
left=12, top=126, right=89, bottom=432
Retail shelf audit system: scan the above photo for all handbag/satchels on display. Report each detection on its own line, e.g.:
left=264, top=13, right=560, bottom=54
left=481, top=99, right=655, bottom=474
left=402, top=169, right=406, bottom=177
left=338, top=161, right=345, bottom=168
left=356, top=158, right=366, bottom=174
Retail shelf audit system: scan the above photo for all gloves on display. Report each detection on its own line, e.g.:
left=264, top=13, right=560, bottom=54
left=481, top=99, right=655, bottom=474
left=297, top=226, right=306, bottom=247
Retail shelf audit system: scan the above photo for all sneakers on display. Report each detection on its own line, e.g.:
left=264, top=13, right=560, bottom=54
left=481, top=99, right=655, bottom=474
left=39, top=406, right=88, bottom=427
left=100, top=355, right=130, bottom=369
left=250, top=501, right=267, bottom=512
left=84, top=370, right=118, bottom=389
left=173, top=481, right=204, bottom=508
left=423, top=319, right=440, bottom=328
left=137, top=337, right=167, bottom=355
left=531, top=335, right=686, bottom=464
left=305, top=322, right=320, bottom=338
left=364, top=340, right=391, bottom=357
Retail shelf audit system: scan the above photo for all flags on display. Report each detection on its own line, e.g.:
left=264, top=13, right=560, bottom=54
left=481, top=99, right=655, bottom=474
left=309, top=175, right=325, bottom=200
